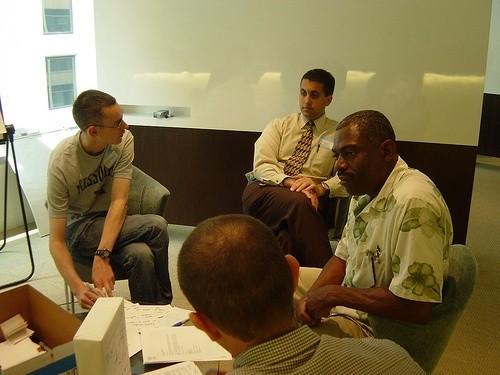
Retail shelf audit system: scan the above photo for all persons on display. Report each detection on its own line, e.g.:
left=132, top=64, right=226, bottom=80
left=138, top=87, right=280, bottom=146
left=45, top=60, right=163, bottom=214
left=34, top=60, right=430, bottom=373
left=241, top=69, right=349, bottom=268
left=47, top=89, right=173, bottom=309
left=177, top=215, right=428, bottom=374
left=297, top=110, right=453, bottom=337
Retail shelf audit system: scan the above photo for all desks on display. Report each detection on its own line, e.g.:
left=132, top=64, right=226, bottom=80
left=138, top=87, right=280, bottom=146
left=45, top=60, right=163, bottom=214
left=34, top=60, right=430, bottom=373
left=74, top=312, right=342, bottom=374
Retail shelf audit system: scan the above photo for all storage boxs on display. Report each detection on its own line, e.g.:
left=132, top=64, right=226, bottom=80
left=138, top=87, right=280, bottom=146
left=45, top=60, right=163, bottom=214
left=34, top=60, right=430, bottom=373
left=0, top=283, right=83, bottom=374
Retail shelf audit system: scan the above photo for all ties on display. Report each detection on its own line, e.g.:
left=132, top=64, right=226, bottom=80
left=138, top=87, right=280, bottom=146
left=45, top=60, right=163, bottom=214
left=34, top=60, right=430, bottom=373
left=283, top=121, right=315, bottom=177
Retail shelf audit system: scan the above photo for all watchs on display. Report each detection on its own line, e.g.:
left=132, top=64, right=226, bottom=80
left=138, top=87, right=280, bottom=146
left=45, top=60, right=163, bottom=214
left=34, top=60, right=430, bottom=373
left=321, top=182, right=331, bottom=196
left=94, top=248, right=111, bottom=258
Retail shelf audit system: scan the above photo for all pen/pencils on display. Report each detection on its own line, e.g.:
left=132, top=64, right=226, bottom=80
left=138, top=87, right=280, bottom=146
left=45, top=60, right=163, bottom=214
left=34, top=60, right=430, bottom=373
left=172, top=317, right=190, bottom=326
left=85, top=282, right=104, bottom=297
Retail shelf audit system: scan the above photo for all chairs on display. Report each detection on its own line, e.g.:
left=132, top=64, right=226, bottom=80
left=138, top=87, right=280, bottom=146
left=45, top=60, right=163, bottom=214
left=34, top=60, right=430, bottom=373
left=44, top=163, right=171, bottom=314
left=243, top=168, right=348, bottom=241
left=367, top=242, right=480, bottom=374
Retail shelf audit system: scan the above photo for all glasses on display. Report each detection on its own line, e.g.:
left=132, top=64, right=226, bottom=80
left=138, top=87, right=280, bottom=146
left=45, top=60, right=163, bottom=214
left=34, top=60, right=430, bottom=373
left=85, top=115, right=123, bottom=129
